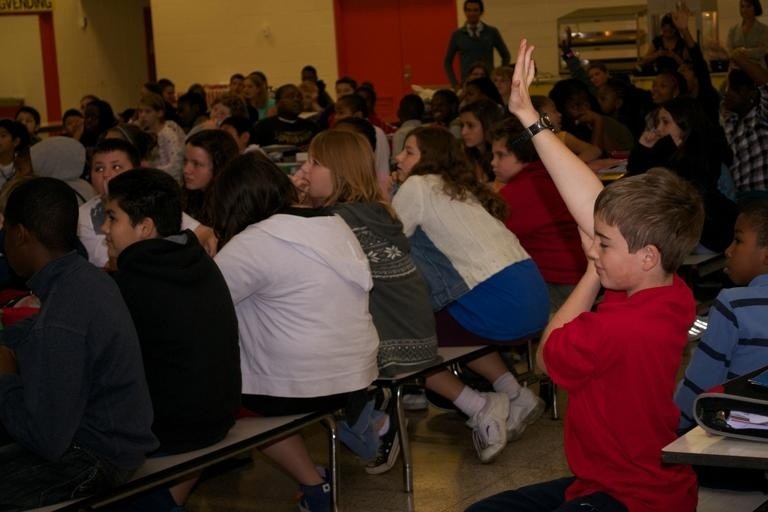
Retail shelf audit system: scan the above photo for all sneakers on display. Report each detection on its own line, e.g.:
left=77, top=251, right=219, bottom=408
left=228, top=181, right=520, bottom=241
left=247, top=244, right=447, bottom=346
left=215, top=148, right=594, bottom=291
left=467, top=388, right=545, bottom=462
left=363, top=415, right=409, bottom=474
left=295, top=465, right=334, bottom=512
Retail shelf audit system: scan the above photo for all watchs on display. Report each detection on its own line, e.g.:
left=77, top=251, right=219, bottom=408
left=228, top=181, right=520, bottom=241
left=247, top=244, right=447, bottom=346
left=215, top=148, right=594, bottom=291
left=525, top=112, right=553, bottom=138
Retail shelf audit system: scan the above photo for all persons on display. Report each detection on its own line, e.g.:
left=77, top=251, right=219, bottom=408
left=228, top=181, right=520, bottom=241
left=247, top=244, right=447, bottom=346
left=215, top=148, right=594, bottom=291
left=468, top=38, right=701, bottom=512
left=445, top=0, right=511, bottom=87
left=0, top=62, right=556, bottom=512
left=557, top=2, right=768, bottom=435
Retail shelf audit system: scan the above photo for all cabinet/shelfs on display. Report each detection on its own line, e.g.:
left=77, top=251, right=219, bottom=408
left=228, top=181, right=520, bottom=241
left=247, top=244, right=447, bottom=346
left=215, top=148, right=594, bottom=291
left=556, top=4, right=650, bottom=74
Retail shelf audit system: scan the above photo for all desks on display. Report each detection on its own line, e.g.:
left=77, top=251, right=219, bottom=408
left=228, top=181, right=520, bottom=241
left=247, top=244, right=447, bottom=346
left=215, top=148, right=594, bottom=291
left=660, top=424, right=768, bottom=470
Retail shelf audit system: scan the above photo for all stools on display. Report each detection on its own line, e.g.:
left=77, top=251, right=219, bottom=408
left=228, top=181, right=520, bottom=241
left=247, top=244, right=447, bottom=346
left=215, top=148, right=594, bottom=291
left=698, top=483, right=768, bottom=512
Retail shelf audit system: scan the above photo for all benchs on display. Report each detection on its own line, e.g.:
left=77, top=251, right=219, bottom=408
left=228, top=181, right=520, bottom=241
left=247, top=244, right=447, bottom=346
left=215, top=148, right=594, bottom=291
left=1, top=338, right=380, bottom=512
left=332, top=341, right=557, bottom=493
